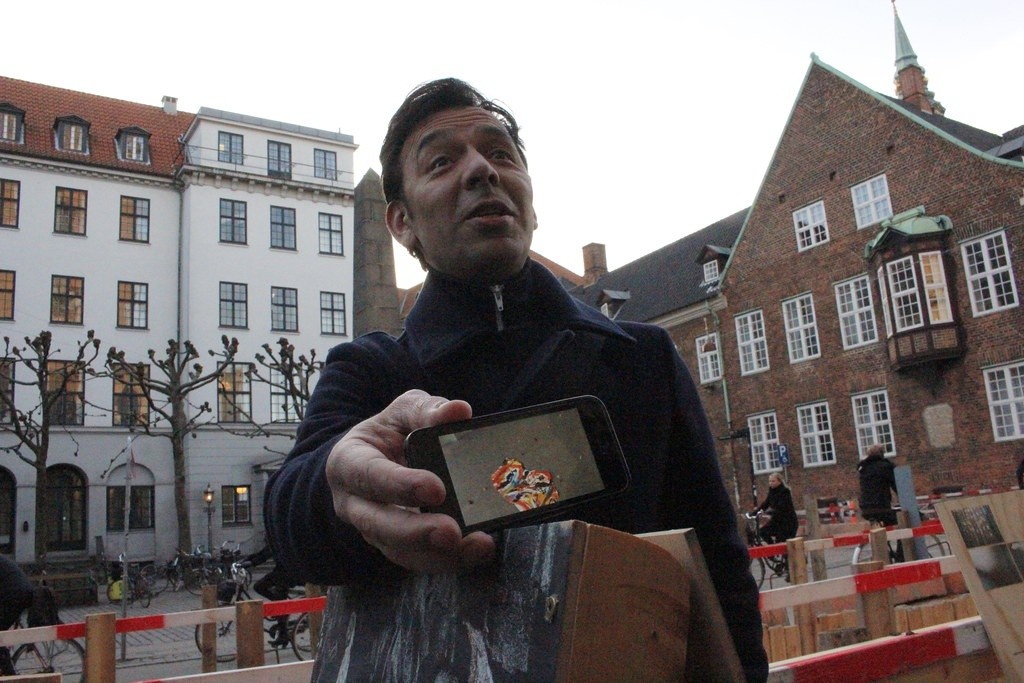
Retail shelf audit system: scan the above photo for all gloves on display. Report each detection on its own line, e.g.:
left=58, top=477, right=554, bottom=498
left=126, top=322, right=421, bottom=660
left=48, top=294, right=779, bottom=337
left=237, top=557, right=248, bottom=564
left=241, top=561, right=252, bottom=569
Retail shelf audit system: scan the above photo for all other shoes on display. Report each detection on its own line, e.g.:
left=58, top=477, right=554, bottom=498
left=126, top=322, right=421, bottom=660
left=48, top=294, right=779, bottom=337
left=768, top=553, right=779, bottom=559
left=785, top=575, right=790, bottom=582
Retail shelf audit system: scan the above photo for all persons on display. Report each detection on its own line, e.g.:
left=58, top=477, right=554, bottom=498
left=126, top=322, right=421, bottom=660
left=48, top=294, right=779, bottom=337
left=747, top=471, right=799, bottom=583
left=0, top=552, right=46, bottom=676
left=240, top=541, right=301, bottom=650
left=855, top=445, right=904, bottom=562
left=263, top=76, right=769, bottom=682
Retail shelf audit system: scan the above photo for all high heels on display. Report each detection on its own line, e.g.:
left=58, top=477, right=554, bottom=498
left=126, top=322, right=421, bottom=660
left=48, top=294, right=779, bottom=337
left=267, top=630, right=290, bottom=649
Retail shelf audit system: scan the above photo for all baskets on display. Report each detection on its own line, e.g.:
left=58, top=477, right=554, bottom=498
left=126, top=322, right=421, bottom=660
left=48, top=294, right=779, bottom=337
left=216, top=578, right=244, bottom=602
left=220, top=550, right=235, bottom=564
left=191, top=557, right=204, bottom=569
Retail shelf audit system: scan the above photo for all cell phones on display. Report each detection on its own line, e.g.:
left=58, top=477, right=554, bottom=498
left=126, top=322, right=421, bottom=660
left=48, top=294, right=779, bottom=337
left=404, top=394, right=634, bottom=540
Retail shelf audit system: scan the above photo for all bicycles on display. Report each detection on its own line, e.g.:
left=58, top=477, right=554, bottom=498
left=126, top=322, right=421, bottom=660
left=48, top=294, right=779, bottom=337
left=0, top=614, right=87, bottom=683
left=745, top=510, right=807, bottom=590
left=853, top=505, right=945, bottom=576
left=195, top=560, right=323, bottom=663
left=102, top=542, right=240, bottom=606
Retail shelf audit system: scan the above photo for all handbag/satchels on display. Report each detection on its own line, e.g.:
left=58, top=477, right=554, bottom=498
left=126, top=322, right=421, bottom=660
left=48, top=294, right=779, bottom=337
left=737, top=520, right=754, bottom=546
left=27, top=586, right=59, bottom=628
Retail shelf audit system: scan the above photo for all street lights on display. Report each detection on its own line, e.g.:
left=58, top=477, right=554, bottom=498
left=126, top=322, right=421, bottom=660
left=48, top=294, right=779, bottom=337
left=201, top=482, right=216, bottom=552
